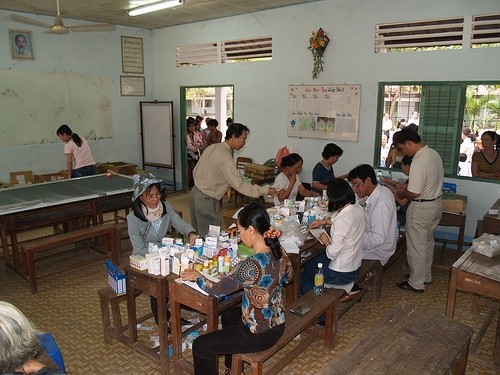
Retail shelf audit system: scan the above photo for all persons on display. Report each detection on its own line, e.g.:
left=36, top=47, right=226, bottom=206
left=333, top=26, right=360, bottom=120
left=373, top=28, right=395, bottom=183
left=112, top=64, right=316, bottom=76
left=189, top=123, right=279, bottom=237
left=386, top=111, right=419, bottom=167
left=193, top=204, right=294, bottom=375
left=0, top=301, right=66, bottom=375
left=274, top=153, right=320, bottom=207
left=127, top=170, right=197, bottom=334
left=459, top=125, right=500, bottom=178
left=187, top=115, right=234, bottom=170
left=381, top=135, right=390, bottom=166
left=299, top=178, right=364, bottom=325
left=383, top=113, right=392, bottom=139
left=383, top=155, right=413, bottom=226
left=56, top=125, right=95, bottom=179
left=348, top=164, right=398, bottom=266
left=312, top=143, right=349, bottom=196
left=394, top=126, right=444, bottom=293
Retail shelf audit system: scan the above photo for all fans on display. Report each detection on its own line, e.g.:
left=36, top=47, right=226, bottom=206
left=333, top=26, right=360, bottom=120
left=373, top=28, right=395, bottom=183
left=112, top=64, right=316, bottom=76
left=10, top=0, right=116, bottom=34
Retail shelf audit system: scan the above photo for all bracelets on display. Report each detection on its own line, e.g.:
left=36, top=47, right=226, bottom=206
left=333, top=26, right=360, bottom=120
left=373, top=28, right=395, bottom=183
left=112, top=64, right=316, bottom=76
left=326, top=241, right=329, bottom=243
left=326, top=221, right=327, bottom=224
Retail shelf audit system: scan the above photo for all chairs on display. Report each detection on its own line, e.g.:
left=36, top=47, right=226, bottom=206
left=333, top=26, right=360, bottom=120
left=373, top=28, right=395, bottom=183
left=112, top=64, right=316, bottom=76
left=228, top=157, right=252, bottom=203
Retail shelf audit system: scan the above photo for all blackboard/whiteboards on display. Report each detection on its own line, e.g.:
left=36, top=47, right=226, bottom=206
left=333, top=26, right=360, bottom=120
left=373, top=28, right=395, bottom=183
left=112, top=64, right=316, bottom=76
left=286, top=84, right=360, bottom=142
left=141, top=103, right=172, bottom=165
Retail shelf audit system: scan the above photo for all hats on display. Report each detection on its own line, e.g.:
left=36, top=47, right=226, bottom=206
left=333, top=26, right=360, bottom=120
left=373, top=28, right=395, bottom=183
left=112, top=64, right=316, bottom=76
left=132, top=172, right=164, bottom=202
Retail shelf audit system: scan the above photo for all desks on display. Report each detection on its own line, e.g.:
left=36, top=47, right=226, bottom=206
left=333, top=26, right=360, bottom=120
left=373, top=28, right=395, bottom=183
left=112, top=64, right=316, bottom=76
left=0, top=175, right=500, bottom=375
left=108, top=163, right=136, bottom=174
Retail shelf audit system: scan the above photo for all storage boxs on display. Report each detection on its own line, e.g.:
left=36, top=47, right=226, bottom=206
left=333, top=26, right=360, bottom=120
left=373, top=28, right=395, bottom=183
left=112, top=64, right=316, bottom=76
left=244, top=165, right=274, bottom=179
left=442, top=195, right=467, bottom=212
left=11, top=171, right=33, bottom=184
left=473, top=235, right=500, bottom=258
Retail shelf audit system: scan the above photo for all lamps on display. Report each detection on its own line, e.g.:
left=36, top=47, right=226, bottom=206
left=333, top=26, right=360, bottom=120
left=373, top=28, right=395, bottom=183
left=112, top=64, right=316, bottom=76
left=126, top=0, right=184, bottom=17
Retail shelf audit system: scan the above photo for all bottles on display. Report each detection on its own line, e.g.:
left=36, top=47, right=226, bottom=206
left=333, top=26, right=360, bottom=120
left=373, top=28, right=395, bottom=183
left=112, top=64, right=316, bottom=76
left=314, top=263, right=324, bottom=296
left=388, top=171, right=392, bottom=180
left=377, top=169, right=383, bottom=180
left=202, top=241, right=234, bottom=276
left=274, top=164, right=278, bottom=177
left=319, top=189, right=328, bottom=220
left=288, top=198, right=295, bottom=209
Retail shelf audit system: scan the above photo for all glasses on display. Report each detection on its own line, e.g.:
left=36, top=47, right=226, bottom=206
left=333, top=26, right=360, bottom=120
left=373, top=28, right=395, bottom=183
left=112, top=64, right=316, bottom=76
left=142, top=193, right=161, bottom=200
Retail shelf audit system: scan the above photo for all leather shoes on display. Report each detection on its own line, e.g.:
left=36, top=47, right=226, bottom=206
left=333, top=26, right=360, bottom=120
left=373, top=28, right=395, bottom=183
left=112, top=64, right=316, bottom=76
left=338, top=291, right=361, bottom=302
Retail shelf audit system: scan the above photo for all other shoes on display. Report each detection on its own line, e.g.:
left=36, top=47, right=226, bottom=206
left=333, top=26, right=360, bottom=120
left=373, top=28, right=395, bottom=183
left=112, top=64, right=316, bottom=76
left=181, top=318, right=191, bottom=324
left=396, top=281, right=424, bottom=293
left=317, top=321, right=325, bottom=326
left=167, top=328, right=173, bottom=334
left=225, top=369, right=244, bottom=375
left=405, top=274, right=431, bottom=284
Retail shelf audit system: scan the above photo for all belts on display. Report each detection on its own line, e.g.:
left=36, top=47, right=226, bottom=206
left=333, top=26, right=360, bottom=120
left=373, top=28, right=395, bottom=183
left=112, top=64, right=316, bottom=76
left=409, top=196, right=441, bottom=202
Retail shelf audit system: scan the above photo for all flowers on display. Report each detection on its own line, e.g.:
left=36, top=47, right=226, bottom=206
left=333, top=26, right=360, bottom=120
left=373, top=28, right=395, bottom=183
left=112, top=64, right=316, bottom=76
left=307, top=30, right=328, bottom=80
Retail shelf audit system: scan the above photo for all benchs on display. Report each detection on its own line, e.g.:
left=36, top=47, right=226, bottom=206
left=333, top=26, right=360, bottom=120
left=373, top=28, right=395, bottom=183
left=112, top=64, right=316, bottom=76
left=99, top=284, right=140, bottom=344
left=113, top=211, right=183, bottom=264
left=20, top=224, right=118, bottom=293
left=359, top=235, right=405, bottom=300
left=304, top=257, right=380, bottom=339
left=230, top=290, right=334, bottom=375
